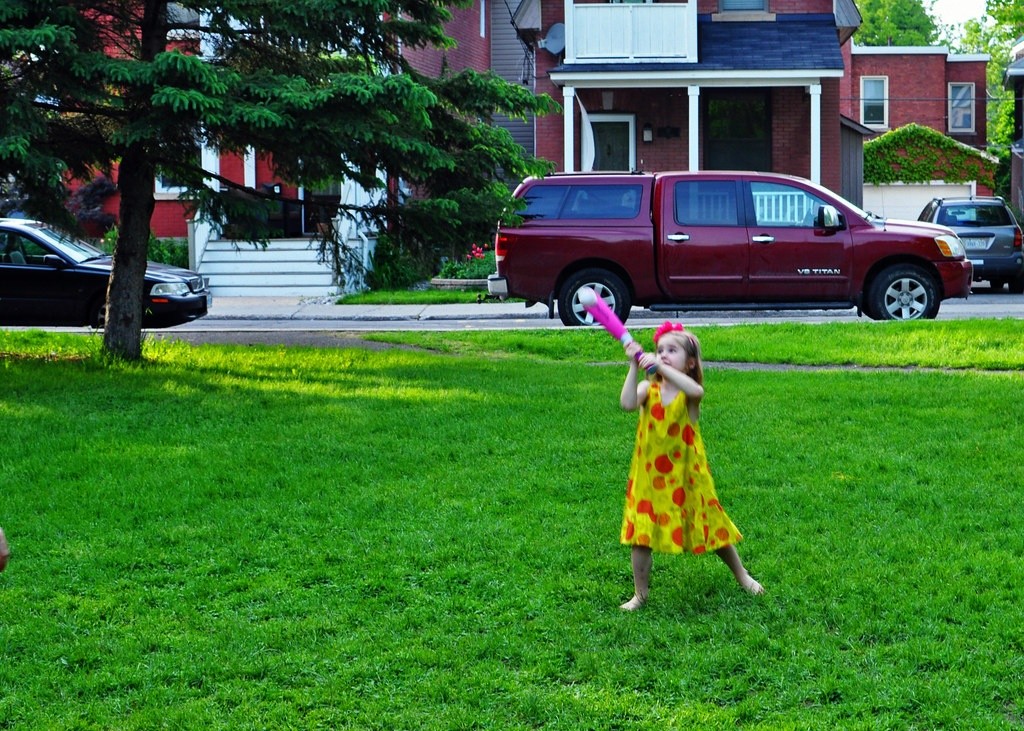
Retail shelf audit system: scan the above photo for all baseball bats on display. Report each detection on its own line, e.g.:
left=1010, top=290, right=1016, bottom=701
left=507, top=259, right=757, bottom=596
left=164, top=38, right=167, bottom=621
left=582, top=292, right=658, bottom=375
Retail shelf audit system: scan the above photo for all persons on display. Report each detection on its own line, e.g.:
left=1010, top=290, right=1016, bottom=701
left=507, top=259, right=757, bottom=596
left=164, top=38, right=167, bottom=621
left=619, top=320, right=764, bottom=612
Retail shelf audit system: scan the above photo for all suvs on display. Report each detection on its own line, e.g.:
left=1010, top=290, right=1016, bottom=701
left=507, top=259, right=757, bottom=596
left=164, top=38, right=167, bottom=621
left=917, top=195, right=1024, bottom=294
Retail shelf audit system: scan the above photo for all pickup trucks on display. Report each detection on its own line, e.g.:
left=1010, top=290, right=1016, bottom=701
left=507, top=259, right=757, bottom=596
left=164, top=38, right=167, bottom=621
left=486, top=170, right=975, bottom=327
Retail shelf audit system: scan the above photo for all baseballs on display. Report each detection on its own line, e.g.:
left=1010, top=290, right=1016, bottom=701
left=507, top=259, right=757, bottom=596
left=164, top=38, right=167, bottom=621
left=577, top=286, right=597, bottom=307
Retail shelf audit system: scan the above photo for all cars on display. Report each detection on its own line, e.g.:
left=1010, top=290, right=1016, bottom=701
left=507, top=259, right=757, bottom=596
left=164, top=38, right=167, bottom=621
left=0, top=217, right=214, bottom=329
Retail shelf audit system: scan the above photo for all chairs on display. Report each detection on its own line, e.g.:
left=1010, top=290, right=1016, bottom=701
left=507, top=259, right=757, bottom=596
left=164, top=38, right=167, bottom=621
left=976, top=210, right=990, bottom=222
left=3, top=237, right=30, bottom=265
left=944, top=214, right=957, bottom=222
